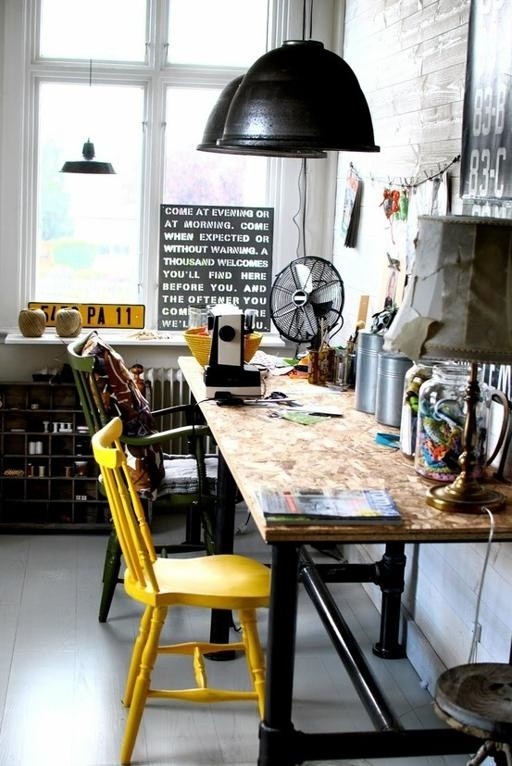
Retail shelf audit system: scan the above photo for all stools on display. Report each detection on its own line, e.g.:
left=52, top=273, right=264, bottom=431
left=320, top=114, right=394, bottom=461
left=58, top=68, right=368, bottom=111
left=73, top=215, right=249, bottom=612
left=432, top=663, right=511, bottom=766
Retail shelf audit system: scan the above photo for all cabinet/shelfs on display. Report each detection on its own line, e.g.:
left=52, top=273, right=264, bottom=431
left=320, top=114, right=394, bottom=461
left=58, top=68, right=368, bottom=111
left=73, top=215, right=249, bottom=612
left=0, top=380, right=114, bottom=530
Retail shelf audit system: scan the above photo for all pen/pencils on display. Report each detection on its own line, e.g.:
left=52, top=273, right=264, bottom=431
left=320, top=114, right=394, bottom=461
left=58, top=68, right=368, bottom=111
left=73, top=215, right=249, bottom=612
left=309, top=412, right=344, bottom=418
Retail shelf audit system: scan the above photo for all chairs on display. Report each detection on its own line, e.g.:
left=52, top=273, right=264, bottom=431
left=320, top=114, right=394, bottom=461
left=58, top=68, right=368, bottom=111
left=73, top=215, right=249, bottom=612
left=66, top=330, right=217, bottom=623
left=91, top=417, right=271, bottom=766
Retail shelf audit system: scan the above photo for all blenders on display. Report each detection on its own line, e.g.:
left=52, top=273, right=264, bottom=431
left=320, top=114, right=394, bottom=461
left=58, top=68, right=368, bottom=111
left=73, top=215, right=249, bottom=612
left=182, top=303, right=262, bottom=399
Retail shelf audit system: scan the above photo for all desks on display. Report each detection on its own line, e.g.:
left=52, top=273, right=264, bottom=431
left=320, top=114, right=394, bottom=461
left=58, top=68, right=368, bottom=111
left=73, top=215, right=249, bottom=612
left=176, top=356, right=512, bottom=766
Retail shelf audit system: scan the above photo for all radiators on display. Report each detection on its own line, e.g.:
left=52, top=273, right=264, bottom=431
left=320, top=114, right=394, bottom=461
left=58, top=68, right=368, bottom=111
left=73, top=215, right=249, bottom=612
left=139, top=367, right=218, bottom=455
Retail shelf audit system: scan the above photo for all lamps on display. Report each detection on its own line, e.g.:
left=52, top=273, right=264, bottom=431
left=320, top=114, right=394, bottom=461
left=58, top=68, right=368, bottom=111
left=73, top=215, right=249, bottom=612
left=216, top=2, right=381, bottom=152
left=57, top=60, right=116, bottom=174
left=382, top=214, right=512, bottom=515
left=196, top=1, right=327, bottom=159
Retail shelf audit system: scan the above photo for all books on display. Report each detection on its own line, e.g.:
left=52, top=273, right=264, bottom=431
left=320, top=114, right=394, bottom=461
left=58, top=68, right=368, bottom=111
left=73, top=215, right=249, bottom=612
left=253, top=486, right=404, bottom=529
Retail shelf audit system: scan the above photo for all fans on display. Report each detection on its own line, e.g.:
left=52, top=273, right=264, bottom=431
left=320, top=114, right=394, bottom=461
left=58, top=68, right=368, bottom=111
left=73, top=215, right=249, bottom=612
left=269, top=255, right=344, bottom=374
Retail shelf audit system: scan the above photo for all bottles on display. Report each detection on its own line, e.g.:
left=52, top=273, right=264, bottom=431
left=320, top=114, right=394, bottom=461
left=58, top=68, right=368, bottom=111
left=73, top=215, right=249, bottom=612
left=414, top=365, right=491, bottom=482
left=354, top=328, right=384, bottom=415
left=399, top=361, right=457, bottom=457
left=375, top=350, right=413, bottom=428
